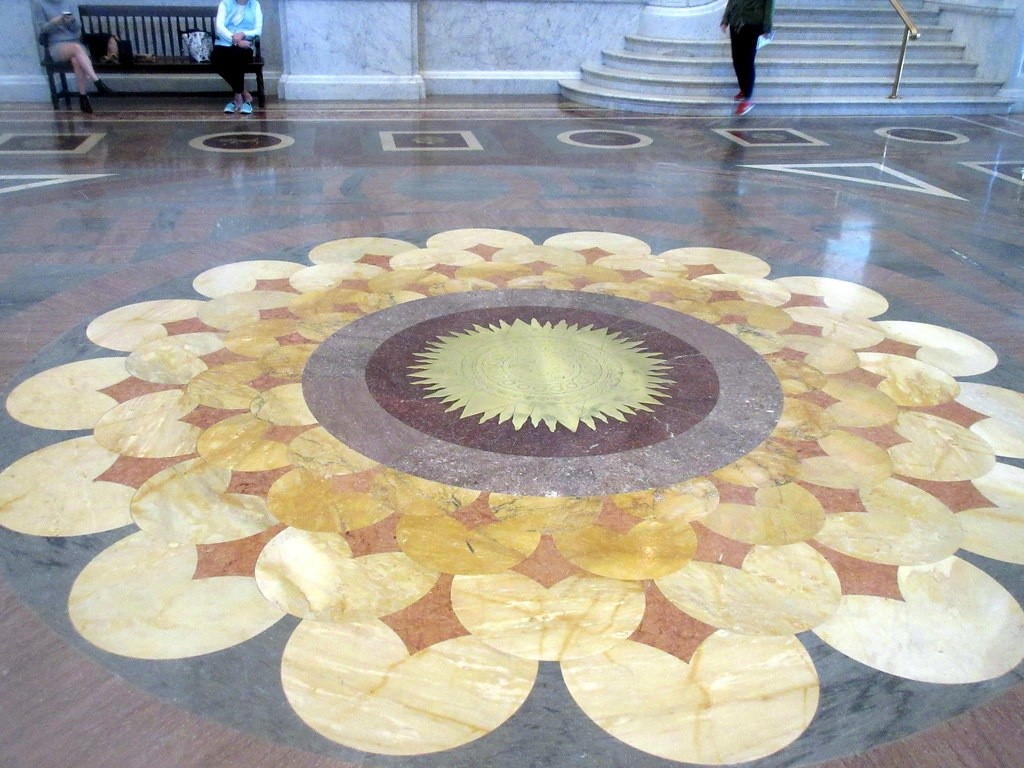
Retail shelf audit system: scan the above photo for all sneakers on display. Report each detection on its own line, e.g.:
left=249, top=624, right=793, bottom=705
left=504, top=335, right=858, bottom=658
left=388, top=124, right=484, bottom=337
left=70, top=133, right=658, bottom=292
left=224, top=102, right=235, bottom=113
left=736, top=99, right=754, bottom=116
left=240, top=102, right=252, bottom=113
left=735, top=92, right=751, bottom=100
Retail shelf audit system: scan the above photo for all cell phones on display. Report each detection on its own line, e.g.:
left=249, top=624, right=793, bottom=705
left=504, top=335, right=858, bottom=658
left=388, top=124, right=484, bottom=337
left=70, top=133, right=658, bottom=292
left=62, top=11, right=73, bottom=17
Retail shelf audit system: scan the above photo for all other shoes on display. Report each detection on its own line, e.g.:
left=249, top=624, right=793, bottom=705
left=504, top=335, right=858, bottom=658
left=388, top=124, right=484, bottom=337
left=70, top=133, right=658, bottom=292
left=94, top=79, right=115, bottom=96
left=80, top=94, right=93, bottom=113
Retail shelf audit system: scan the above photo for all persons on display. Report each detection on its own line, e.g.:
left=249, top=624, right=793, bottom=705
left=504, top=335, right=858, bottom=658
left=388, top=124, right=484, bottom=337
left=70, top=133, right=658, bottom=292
left=210, top=0, right=263, bottom=109
left=32, top=0, right=113, bottom=113
left=721, top=0, right=775, bottom=116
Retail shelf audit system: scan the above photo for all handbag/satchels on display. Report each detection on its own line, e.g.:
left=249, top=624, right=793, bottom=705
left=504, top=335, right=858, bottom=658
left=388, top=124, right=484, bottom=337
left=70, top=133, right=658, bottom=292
left=181, top=29, right=214, bottom=62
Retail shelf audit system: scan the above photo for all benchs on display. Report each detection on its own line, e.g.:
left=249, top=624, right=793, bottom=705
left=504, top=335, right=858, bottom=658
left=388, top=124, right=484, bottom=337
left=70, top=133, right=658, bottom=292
left=39, top=4, right=265, bottom=109
